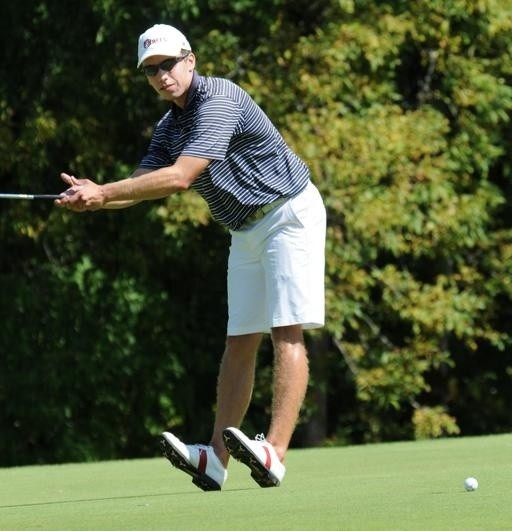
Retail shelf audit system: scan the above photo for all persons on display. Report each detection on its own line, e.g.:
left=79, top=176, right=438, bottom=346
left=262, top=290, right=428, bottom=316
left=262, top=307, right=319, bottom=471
left=54, top=21, right=328, bottom=493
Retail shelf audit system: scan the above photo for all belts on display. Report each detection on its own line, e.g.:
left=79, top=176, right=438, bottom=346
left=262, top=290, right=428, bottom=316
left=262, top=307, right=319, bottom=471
left=242, top=195, right=285, bottom=229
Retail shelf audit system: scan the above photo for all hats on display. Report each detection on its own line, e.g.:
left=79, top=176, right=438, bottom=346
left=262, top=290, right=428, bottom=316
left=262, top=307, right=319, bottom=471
left=137, top=24, right=191, bottom=68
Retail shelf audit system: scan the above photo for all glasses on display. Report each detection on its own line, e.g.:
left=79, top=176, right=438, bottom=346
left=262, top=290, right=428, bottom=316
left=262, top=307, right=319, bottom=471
left=141, top=53, right=188, bottom=76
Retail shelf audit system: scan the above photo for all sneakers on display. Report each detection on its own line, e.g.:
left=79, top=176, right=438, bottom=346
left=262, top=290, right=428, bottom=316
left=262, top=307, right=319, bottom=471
left=223, top=427, right=286, bottom=487
left=160, top=431, right=227, bottom=491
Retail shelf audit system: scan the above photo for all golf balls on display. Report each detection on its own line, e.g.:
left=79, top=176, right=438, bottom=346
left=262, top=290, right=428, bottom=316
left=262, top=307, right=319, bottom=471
left=464, top=477, right=478, bottom=491
